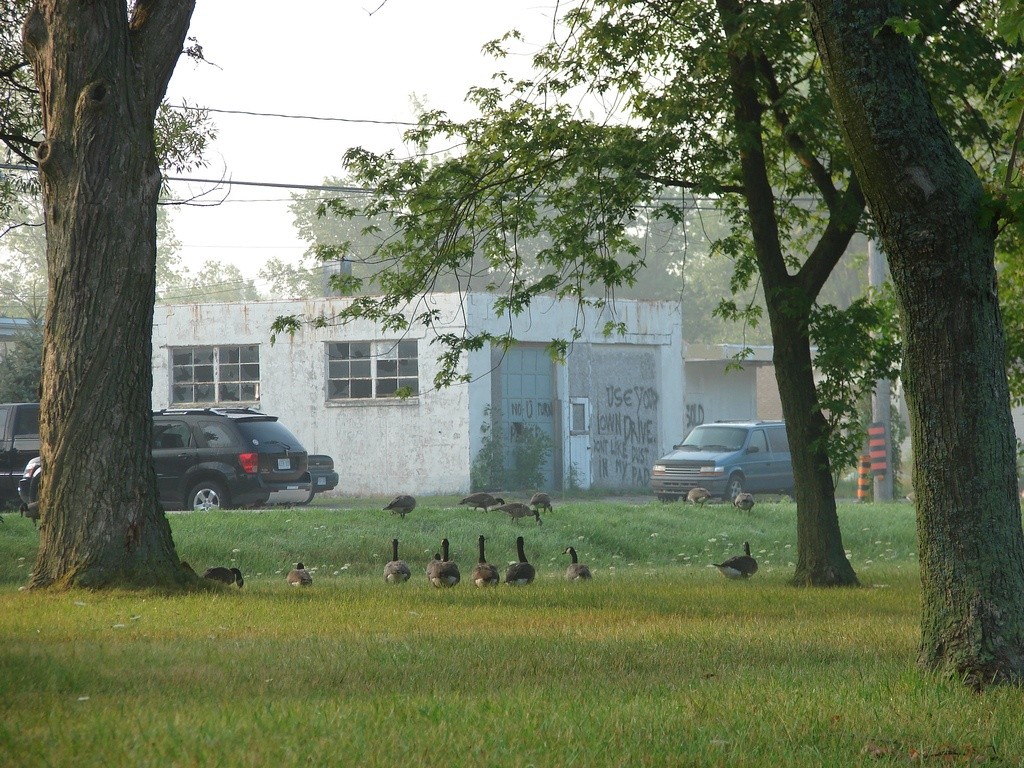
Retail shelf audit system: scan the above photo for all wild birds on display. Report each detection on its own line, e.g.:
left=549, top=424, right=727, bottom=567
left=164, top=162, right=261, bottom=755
left=472, top=534, right=499, bottom=587
left=430, top=540, right=460, bottom=588
left=687, top=488, right=712, bottom=506
left=286, top=563, right=312, bottom=587
left=490, top=503, right=542, bottom=527
left=712, top=541, right=758, bottom=580
left=202, top=567, right=244, bottom=589
left=427, top=554, right=442, bottom=580
left=384, top=539, right=411, bottom=583
left=735, top=494, right=756, bottom=513
left=382, top=495, right=416, bottom=518
left=503, top=536, right=535, bottom=585
left=457, top=492, right=505, bottom=514
left=562, top=547, right=591, bottom=581
left=529, top=492, right=553, bottom=513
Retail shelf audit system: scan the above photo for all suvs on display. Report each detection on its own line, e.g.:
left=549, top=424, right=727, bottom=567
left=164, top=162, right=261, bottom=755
left=0, top=402, right=44, bottom=505
left=648, top=420, right=796, bottom=504
left=17, top=407, right=307, bottom=512
